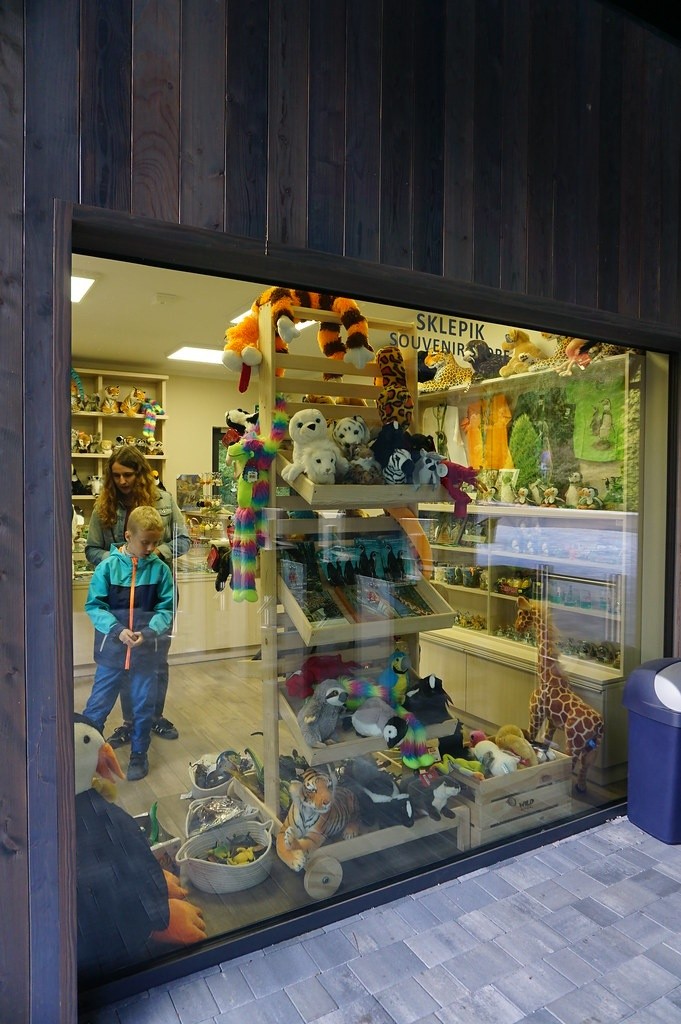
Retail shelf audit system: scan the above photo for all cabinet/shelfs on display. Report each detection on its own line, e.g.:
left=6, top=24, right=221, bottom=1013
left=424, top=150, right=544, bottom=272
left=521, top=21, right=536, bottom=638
left=233, top=299, right=471, bottom=899
left=420, top=354, right=646, bottom=787
left=70, top=368, right=169, bottom=561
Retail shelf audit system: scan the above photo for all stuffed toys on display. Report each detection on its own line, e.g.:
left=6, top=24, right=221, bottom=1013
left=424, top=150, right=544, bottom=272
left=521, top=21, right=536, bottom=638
left=206, top=286, right=488, bottom=601
left=191, top=649, right=556, bottom=873
left=514, top=596, right=605, bottom=792
left=72, top=711, right=209, bottom=985
left=69, top=380, right=164, bottom=551
left=485, top=472, right=618, bottom=510
left=416, top=329, right=644, bottom=397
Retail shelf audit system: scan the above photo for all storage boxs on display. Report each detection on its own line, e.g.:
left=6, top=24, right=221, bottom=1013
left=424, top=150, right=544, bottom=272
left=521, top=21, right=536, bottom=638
left=133, top=812, right=181, bottom=879
left=445, top=734, right=572, bottom=849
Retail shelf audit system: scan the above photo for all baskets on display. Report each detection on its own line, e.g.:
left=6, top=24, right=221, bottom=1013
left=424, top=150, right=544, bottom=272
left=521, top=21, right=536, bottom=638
left=173, top=752, right=277, bottom=894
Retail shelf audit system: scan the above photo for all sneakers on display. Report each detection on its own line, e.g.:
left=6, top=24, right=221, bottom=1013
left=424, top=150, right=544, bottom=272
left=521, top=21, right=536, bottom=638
left=151, top=716, right=178, bottom=739
left=106, top=722, right=135, bottom=748
left=127, top=752, right=149, bottom=781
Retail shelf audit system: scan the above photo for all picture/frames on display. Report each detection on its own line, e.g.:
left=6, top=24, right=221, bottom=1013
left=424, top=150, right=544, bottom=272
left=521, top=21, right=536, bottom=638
left=329, top=582, right=439, bottom=624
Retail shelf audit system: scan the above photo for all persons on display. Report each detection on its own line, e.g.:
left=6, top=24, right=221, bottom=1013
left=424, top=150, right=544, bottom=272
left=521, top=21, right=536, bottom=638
left=82, top=506, right=174, bottom=781
left=85, top=445, right=191, bottom=749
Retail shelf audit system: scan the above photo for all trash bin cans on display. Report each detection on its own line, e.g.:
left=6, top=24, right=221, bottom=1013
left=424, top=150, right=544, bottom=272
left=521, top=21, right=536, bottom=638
left=618, top=658, right=681, bottom=845
